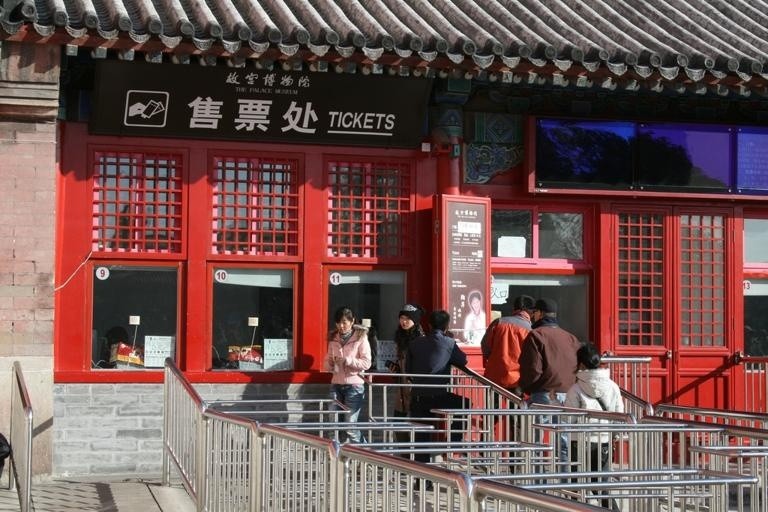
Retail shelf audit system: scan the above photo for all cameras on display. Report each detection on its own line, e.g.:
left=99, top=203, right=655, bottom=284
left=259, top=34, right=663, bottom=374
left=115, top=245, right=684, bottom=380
left=385, top=359, right=399, bottom=373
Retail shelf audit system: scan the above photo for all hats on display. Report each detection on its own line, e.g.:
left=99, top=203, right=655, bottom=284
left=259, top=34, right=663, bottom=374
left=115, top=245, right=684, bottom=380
left=535, top=298, right=558, bottom=313
left=399, top=303, right=420, bottom=324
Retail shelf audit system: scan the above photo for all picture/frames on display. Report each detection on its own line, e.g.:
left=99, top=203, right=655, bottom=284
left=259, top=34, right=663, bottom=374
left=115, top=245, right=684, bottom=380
left=440, top=194, right=491, bottom=355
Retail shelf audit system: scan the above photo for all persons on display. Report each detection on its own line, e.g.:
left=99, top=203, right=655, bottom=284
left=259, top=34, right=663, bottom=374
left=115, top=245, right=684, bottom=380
left=323, top=308, right=372, bottom=443
left=105, top=326, right=129, bottom=350
left=388, top=303, right=426, bottom=457
left=566, top=346, right=625, bottom=512
left=405, top=310, right=469, bottom=490
left=481, top=295, right=536, bottom=474
left=518, top=297, right=582, bottom=493
